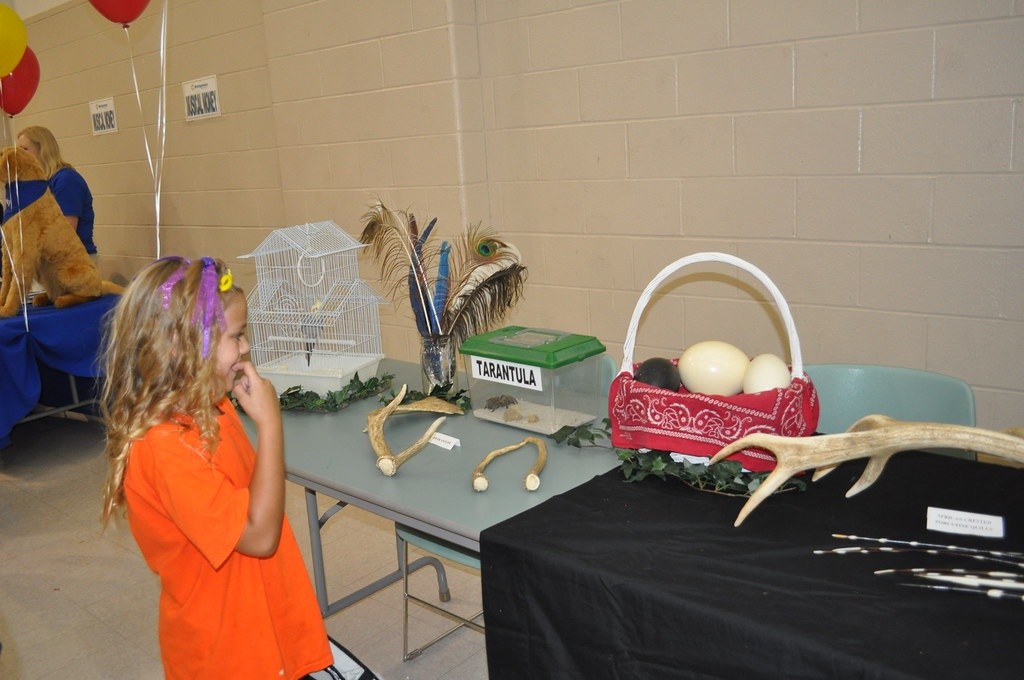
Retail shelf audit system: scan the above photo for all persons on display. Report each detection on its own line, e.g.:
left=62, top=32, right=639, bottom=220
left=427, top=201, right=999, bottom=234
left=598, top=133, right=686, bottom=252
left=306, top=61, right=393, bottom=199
left=17, top=127, right=98, bottom=267
left=98, top=255, right=334, bottom=680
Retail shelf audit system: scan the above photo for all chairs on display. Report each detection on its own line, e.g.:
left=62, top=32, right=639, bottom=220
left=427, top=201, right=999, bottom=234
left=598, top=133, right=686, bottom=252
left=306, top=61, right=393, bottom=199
left=394, top=356, right=616, bottom=663
left=803, top=364, right=976, bottom=462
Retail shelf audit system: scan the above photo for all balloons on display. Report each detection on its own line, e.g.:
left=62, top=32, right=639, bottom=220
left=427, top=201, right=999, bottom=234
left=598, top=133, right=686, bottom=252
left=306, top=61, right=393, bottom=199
left=0, top=45, right=40, bottom=118
left=90, top=0, right=150, bottom=28
left=0, top=0, right=27, bottom=78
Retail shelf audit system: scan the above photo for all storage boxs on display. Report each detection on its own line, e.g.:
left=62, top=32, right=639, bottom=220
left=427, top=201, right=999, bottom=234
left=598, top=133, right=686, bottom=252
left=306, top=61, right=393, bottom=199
left=459, top=326, right=606, bottom=435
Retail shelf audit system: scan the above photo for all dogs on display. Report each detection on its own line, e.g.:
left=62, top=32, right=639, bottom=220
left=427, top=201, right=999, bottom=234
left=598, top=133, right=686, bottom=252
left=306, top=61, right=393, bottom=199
left=0, top=145, right=129, bottom=318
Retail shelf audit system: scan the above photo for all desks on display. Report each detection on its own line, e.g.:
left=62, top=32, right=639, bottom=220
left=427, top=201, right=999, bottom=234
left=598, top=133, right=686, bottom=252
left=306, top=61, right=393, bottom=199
left=0, top=295, right=120, bottom=449
left=236, top=359, right=1023, bottom=680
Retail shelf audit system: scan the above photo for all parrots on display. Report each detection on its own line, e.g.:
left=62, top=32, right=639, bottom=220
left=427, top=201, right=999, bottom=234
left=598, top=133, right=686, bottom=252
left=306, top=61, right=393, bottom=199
left=300, top=299, right=324, bottom=367
